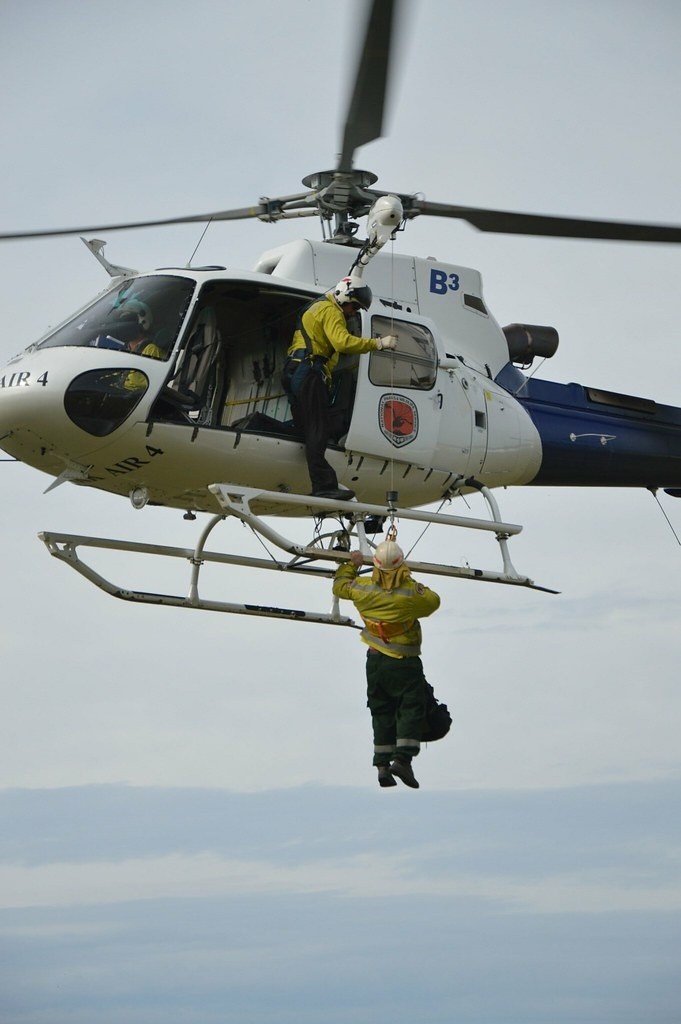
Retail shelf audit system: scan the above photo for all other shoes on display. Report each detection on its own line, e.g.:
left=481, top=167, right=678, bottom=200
left=389, top=758, right=419, bottom=789
left=378, top=765, right=397, bottom=787
left=311, top=483, right=355, bottom=501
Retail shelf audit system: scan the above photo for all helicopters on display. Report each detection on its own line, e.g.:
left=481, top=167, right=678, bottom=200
left=0, top=0, right=680, bottom=633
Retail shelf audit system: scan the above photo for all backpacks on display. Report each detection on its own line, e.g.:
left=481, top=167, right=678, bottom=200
left=414, top=674, right=452, bottom=742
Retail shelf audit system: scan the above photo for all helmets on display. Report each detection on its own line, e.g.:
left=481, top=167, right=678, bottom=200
left=118, top=298, right=153, bottom=331
left=372, top=540, right=404, bottom=571
left=334, top=276, right=373, bottom=312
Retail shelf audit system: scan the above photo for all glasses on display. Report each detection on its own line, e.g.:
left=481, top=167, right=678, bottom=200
left=351, top=302, right=360, bottom=311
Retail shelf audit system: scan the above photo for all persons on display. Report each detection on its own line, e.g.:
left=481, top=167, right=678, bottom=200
left=278, top=273, right=397, bottom=501
left=332, top=541, right=441, bottom=789
left=105, top=297, right=165, bottom=391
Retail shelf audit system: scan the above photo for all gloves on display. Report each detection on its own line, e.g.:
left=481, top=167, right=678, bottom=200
left=381, top=334, right=398, bottom=351
